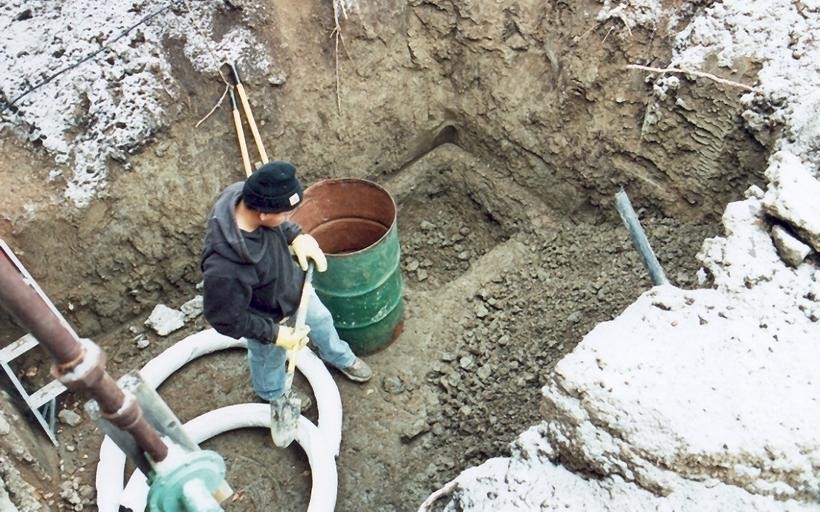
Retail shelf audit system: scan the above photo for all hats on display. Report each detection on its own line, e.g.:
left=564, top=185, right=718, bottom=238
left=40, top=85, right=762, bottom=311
left=242, top=160, right=302, bottom=210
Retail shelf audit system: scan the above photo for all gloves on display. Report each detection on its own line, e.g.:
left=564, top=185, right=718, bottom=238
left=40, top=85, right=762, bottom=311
left=272, top=325, right=311, bottom=352
left=291, top=233, right=328, bottom=273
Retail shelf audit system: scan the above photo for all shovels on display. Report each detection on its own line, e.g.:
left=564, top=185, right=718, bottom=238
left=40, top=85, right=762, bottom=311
left=271, top=258, right=314, bottom=449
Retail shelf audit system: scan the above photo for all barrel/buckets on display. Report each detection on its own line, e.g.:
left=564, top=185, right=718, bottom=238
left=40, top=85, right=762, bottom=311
left=285, top=177, right=405, bottom=357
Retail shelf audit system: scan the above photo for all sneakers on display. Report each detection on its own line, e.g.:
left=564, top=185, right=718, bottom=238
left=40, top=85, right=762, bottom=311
left=257, top=385, right=312, bottom=413
left=319, top=351, right=373, bottom=383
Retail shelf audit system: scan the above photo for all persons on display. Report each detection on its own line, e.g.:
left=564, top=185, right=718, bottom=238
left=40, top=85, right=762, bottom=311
left=200, top=161, right=374, bottom=414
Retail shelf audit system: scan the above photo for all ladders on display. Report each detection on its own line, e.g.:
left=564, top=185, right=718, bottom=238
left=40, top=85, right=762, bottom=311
left=0, top=238, right=80, bottom=448
